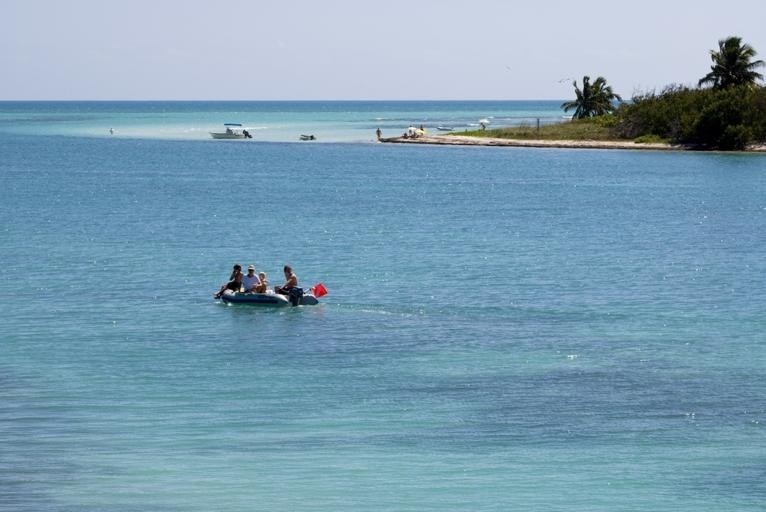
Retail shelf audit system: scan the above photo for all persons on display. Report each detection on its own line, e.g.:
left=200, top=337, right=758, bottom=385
left=376, top=128, right=382, bottom=138
left=274, top=266, right=298, bottom=295
left=241, top=265, right=259, bottom=292
left=253, top=272, right=268, bottom=293
left=480, top=122, right=486, bottom=132
left=213, top=264, right=243, bottom=299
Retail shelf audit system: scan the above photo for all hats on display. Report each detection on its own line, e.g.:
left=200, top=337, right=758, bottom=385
left=248, top=265, right=255, bottom=271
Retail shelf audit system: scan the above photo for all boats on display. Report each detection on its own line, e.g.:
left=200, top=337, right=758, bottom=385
left=221, top=286, right=318, bottom=305
left=210, top=126, right=249, bottom=140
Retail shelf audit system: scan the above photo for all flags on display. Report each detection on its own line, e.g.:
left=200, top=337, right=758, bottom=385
left=310, top=283, right=327, bottom=299
